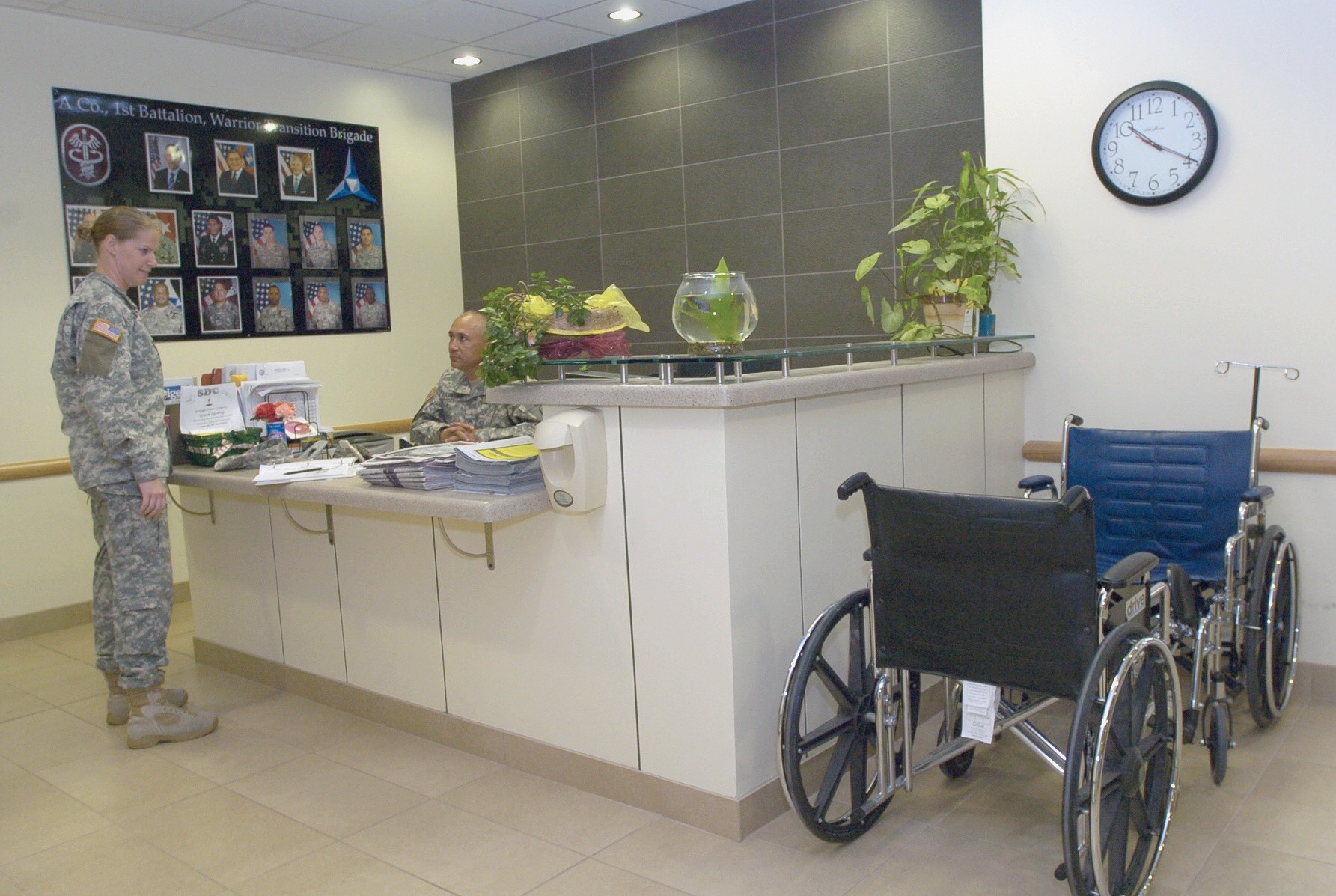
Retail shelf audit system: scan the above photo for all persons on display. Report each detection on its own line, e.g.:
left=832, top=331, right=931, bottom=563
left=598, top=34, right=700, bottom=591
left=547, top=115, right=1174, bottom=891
left=409, top=311, right=544, bottom=444
left=309, top=285, right=342, bottom=330
left=49, top=206, right=218, bottom=751
left=303, top=224, right=338, bottom=269
left=146, top=214, right=179, bottom=264
left=350, top=226, right=383, bottom=269
left=255, top=224, right=289, bottom=268
left=357, top=288, right=387, bottom=328
left=139, top=283, right=182, bottom=334
left=219, top=150, right=256, bottom=195
left=69, top=213, right=99, bottom=263
left=197, top=214, right=234, bottom=265
left=284, top=155, right=314, bottom=197
left=152, top=144, right=190, bottom=192
left=202, top=281, right=239, bottom=331
left=257, top=285, right=293, bottom=332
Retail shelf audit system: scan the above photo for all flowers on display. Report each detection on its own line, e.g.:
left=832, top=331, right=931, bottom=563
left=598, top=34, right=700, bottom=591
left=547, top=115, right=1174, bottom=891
left=249, top=402, right=296, bottom=423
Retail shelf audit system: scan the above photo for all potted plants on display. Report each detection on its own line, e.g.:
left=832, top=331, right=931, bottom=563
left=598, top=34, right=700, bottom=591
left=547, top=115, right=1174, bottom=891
left=477, top=270, right=631, bottom=392
left=855, top=149, right=1046, bottom=342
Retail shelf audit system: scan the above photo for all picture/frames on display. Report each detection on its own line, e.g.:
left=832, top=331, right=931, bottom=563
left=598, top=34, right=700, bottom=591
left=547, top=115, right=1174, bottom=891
left=303, top=277, right=343, bottom=330
left=299, top=215, right=338, bottom=269
left=197, top=276, right=242, bottom=333
left=351, top=278, right=387, bottom=328
left=347, top=217, right=384, bottom=269
left=214, top=139, right=259, bottom=198
left=276, top=146, right=317, bottom=202
left=252, top=278, right=294, bottom=332
left=65, top=204, right=112, bottom=267
left=136, top=208, right=181, bottom=267
left=145, top=133, right=194, bottom=195
left=192, top=210, right=238, bottom=268
left=138, top=277, right=186, bottom=335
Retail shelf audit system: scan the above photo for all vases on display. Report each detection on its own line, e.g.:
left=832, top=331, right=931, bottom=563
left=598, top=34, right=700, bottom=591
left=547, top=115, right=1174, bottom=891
left=267, top=422, right=287, bottom=445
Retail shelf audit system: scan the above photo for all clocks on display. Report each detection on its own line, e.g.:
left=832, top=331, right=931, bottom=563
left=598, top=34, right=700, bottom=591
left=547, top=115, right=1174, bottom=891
left=1091, top=81, right=1219, bottom=206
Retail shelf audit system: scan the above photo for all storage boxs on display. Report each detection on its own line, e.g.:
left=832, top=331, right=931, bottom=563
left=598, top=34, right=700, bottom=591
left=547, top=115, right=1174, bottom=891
left=177, top=427, right=262, bottom=465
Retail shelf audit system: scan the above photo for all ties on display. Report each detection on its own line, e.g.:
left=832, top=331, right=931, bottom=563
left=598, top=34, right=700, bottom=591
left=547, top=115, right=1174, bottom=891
left=212, top=238, right=215, bottom=243
left=232, top=172, right=237, bottom=181
left=168, top=173, right=175, bottom=191
left=294, top=178, right=298, bottom=194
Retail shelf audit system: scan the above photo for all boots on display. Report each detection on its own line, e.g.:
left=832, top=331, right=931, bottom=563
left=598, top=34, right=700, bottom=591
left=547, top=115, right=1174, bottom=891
left=126, top=684, right=216, bottom=749
left=104, top=674, right=188, bottom=726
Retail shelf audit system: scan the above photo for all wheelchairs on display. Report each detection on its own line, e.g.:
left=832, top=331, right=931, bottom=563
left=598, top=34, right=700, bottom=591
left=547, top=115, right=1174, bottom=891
left=1018, top=414, right=1303, bottom=785
left=774, top=469, right=1235, bottom=896
